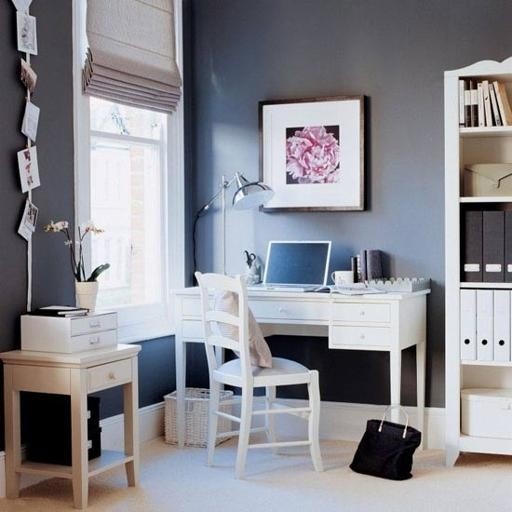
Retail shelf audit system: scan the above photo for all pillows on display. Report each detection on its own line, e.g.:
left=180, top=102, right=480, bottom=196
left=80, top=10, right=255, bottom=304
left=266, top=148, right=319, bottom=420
left=212, top=289, right=272, bottom=369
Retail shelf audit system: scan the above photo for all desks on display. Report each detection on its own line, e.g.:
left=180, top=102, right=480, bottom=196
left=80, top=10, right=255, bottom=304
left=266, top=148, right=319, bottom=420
left=170, top=286, right=431, bottom=453
left=0, top=345, right=142, bottom=508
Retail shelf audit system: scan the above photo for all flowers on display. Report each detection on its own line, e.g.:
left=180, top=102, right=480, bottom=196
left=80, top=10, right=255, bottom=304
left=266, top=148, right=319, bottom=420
left=44, top=219, right=110, bottom=282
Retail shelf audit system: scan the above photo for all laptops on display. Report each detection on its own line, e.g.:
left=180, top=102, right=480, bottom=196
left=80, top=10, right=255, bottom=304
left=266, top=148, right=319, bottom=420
left=248, top=241, right=334, bottom=293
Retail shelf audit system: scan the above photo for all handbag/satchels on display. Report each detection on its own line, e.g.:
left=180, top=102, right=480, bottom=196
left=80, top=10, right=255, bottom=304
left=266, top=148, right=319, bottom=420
left=349, top=419, right=421, bottom=480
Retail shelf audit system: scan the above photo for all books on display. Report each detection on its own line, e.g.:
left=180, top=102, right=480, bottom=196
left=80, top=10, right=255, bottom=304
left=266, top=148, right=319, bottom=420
left=459, top=79, right=512, bottom=127
left=30, top=305, right=90, bottom=317
left=351, top=249, right=383, bottom=283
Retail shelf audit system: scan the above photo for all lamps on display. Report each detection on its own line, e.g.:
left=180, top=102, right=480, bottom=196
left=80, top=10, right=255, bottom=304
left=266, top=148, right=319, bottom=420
left=192, top=173, right=275, bottom=286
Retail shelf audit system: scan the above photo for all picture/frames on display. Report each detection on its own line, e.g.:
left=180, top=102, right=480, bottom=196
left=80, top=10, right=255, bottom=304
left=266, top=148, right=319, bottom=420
left=257, top=95, right=366, bottom=212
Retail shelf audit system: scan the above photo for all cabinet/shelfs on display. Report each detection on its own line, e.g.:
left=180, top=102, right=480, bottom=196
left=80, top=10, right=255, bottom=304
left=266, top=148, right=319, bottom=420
left=442, top=55, right=512, bottom=467
left=21, top=312, right=119, bottom=352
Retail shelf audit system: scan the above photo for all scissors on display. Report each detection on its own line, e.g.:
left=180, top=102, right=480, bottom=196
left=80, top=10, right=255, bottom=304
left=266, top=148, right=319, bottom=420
left=243, top=250, right=256, bottom=269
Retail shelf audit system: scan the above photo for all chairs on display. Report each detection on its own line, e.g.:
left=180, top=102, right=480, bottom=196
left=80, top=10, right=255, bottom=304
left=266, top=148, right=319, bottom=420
left=195, top=272, right=323, bottom=478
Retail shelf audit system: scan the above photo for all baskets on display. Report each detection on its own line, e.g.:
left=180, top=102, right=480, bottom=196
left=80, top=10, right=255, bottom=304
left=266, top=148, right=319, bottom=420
left=163, top=387, right=234, bottom=448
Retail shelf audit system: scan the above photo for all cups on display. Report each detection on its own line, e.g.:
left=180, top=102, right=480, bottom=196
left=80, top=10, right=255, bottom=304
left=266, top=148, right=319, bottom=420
left=331, top=269, right=354, bottom=288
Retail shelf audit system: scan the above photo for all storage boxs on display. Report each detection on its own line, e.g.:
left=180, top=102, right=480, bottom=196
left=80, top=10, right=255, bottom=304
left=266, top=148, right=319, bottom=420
left=40, top=396, right=102, bottom=465
left=460, top=388, right=511, bottom=438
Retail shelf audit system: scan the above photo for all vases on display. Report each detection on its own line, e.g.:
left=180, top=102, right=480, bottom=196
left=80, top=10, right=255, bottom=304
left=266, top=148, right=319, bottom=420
left=75, top=281, right=99, bottom=314
